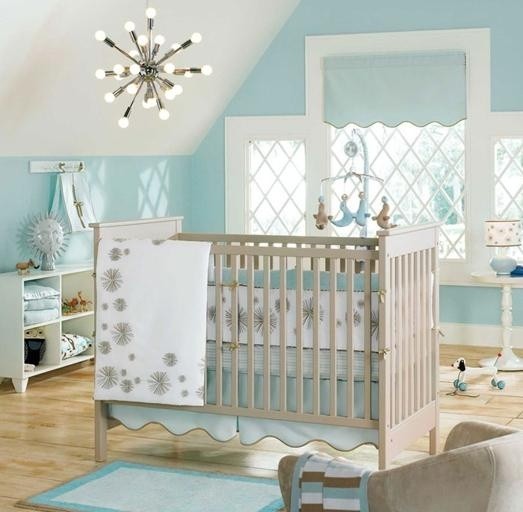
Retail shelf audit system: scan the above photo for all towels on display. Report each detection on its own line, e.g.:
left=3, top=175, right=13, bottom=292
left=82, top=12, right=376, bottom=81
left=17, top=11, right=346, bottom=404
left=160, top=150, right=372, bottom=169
left=290, top=450, right=373, bottom=512
left=49, top=172, right=98, bottom=232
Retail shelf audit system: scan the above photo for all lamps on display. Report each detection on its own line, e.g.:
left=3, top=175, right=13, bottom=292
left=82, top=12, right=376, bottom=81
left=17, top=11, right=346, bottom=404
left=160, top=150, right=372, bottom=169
left=484, top=220, right=523, bottom=275
left=94, top=0, right=212, bottom=128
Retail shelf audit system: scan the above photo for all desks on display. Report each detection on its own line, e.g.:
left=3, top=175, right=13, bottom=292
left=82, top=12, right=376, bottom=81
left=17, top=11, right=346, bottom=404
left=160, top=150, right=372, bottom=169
left=468, top=275, right=523, bottom=371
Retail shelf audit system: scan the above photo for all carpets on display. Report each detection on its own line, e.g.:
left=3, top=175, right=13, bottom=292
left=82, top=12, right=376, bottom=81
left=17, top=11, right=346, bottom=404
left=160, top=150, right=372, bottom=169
left=14, top=459, right=285, bottom=512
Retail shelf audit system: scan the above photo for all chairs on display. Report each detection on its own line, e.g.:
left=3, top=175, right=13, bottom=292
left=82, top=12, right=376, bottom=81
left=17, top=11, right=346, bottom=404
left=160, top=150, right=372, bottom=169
left=278, top=421, right=523, bottom=512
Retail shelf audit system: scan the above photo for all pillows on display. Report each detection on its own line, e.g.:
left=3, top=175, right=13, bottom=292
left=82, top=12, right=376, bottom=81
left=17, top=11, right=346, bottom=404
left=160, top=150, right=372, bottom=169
left=62, top=334, right=93, bottom=360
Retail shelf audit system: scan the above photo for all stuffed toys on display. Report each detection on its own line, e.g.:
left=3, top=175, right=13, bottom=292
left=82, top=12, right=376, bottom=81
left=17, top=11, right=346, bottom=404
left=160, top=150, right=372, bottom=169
left=328, top=194, right=353, bottom=226
left=62, top=290, right=92, bottom=315
left=351, top=192, right=370, bottom=226
left=371, top=196, right=399, bottom=230
left=312, top=196, right=332, bottom=229
left=14, top=258, right=39, bottom=274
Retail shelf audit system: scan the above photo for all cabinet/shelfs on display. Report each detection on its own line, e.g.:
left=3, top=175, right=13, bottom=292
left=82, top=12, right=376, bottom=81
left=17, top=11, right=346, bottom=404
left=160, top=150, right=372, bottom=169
left=0, top=264, right=94, bottom=393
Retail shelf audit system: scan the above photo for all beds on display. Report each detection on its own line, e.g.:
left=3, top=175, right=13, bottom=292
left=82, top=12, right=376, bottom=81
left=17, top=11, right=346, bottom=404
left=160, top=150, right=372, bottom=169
left=89, top=216, right=439, bottom=473
